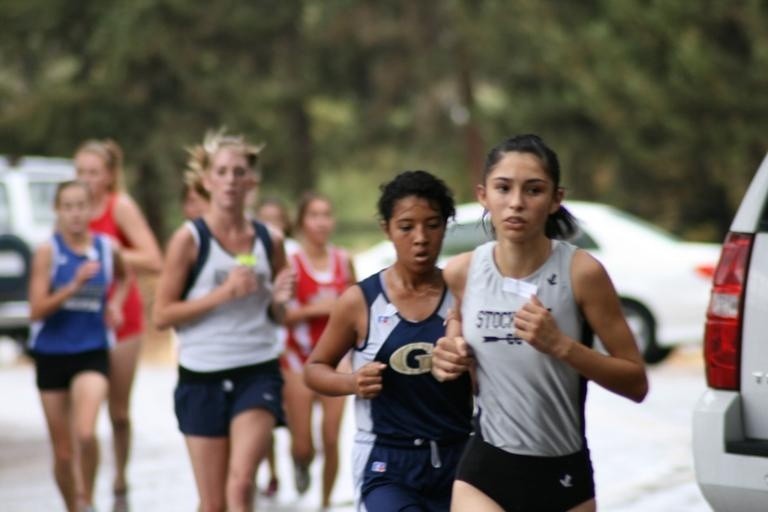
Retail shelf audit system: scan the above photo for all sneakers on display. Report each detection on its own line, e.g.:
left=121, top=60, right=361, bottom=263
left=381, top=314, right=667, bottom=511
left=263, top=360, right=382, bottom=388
left=267, top=479, right=277, bottom=492
left=114, top=489, right=128, bottom=512
left=295, top=450, right=316, bottom=490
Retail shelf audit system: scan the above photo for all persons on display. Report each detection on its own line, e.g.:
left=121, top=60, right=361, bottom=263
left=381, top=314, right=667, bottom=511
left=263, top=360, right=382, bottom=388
left=28, top=181, right=133, bottom=510
left=152, top=126, right=356, bottom=511
left=71, top=137, right=163, bottom=493
left=430, top=133, right=648, bottom=512
left=304, top=169, right=478, bottom=512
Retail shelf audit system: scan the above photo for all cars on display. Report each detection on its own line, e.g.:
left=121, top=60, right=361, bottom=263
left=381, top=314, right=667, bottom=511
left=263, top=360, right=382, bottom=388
left=692, top=154, right=768, bottom=512
left=349, top=199, right=720, bottom=364
left=2, top=155, right=75, bottom=331
